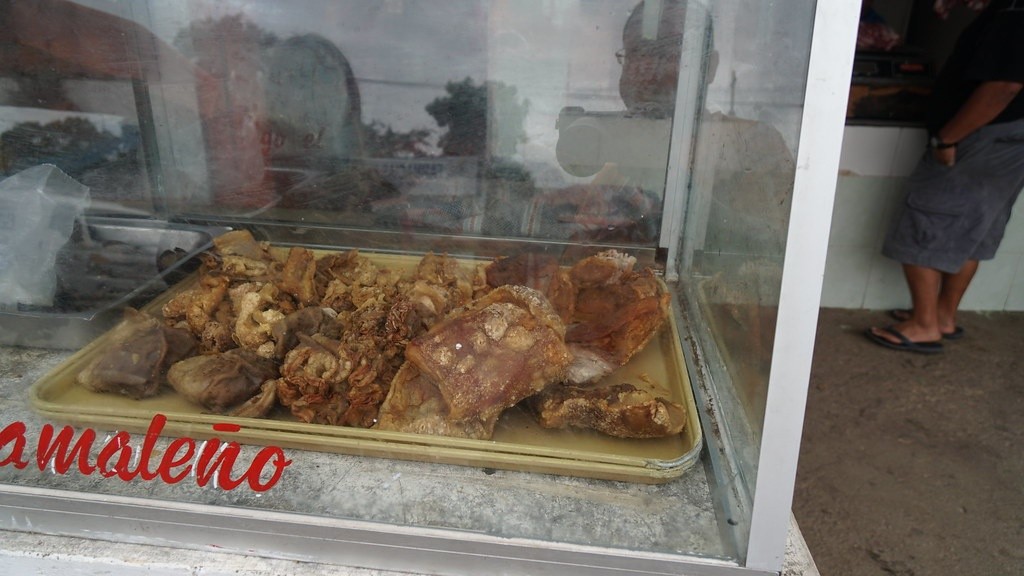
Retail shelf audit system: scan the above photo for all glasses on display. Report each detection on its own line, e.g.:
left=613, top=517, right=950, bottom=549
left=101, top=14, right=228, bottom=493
left=273, top=62, right=356, bottom=93
left=615, top=41, right=717, bottom=73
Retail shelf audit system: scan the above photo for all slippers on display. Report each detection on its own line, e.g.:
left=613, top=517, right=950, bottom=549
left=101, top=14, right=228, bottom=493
left=888, top=307, right=966, bottom=340
left=862, top=325, right=944, bottom=359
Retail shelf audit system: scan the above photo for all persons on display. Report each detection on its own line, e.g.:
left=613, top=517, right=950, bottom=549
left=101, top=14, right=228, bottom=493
left=246, top=35, right=419, bottom=235
left=554, top=0, right=797, bottom=382
left=861, top=1, right=1024, bottom=356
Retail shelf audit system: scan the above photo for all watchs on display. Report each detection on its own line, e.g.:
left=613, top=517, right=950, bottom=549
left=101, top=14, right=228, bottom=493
left=929, top=131, right=957, bottom=149
